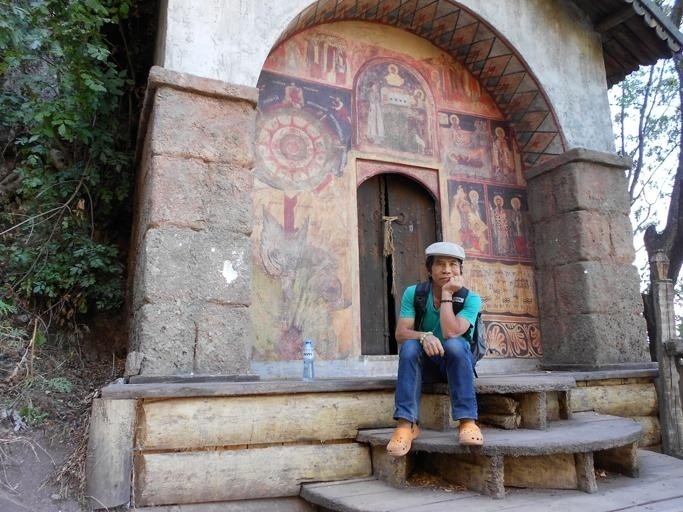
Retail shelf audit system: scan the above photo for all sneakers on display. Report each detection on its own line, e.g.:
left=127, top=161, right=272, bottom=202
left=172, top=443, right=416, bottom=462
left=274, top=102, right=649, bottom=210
left=386, top=423, right=420, bottom=457
left=458, top=421, right=483, bottom=445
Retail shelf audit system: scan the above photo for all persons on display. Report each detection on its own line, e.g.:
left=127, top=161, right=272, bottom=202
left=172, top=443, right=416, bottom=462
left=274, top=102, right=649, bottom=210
left=283, top=80, right=351, bottom=125
left=367, top=82, right=528, bottom=257
left=387, top=243, right=484, bottom=457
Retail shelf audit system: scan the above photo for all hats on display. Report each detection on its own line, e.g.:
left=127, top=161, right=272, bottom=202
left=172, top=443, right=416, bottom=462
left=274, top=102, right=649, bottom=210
left=425, top=242, right=465, bottom=261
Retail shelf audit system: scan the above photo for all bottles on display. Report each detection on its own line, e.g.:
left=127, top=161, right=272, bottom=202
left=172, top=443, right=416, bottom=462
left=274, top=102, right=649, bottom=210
left=302, top=340, right=314, bottom=380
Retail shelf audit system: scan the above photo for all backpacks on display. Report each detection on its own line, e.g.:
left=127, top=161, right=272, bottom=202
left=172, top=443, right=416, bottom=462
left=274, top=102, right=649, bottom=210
left=414, top=280, right=486, bottom=378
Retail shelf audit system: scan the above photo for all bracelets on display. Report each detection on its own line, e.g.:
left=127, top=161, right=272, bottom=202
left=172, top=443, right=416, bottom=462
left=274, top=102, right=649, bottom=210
left=439, top=300, right=453, bottom=303
left=420, top=331, right=433, bottom=345
